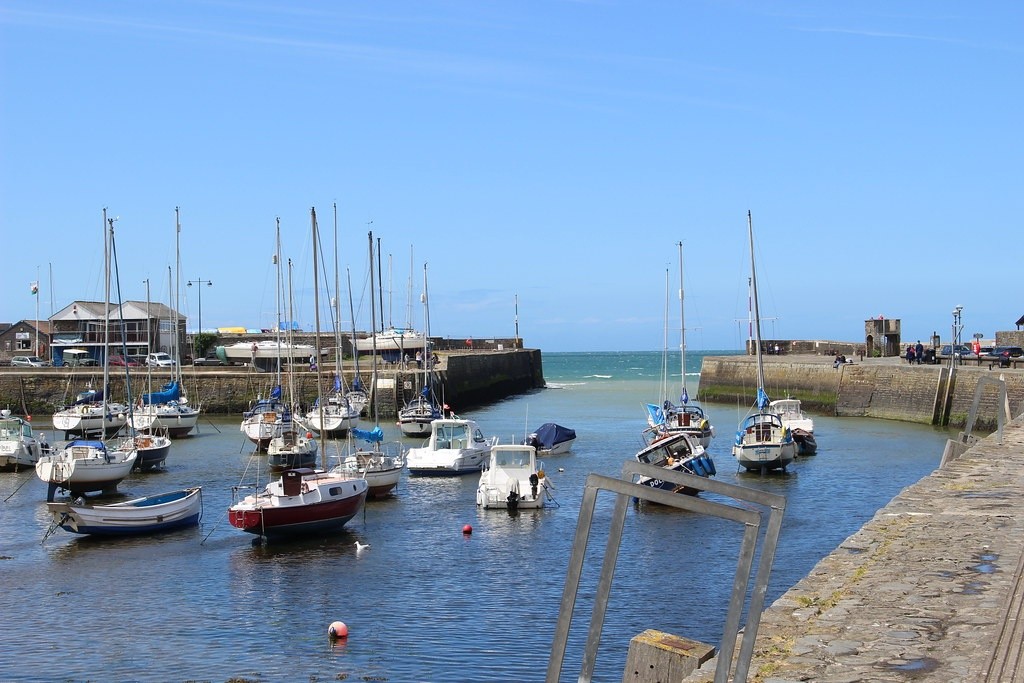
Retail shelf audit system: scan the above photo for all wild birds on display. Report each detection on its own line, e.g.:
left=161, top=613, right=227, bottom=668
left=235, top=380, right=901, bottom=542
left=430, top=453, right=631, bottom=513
left=354, top=541, right=371, bottom=549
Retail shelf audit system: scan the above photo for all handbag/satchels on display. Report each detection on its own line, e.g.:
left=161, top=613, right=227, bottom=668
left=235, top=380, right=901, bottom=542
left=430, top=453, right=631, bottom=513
left=310, top=364, right=317, bottom=369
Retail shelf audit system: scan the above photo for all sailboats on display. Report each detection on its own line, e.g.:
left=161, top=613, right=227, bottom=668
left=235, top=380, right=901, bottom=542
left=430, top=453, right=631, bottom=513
left=732, top=210, right=798, bottom=476
left=217, top=203, right=444, bottom=489
left=35, top=205, right=202, bottom=492
left=648, top=240, right=714, bottom=448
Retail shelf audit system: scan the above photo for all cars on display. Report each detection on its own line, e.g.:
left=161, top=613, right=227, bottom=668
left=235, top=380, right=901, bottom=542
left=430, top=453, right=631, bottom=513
left=11, top=355, right=50, bottom=367
left=979, top=348, right=992, bottom=356
left=109, top=355, right=142, bottom=366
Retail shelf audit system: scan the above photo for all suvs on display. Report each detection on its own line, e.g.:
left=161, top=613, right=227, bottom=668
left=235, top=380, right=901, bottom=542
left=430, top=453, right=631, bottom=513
left=941, top=345, right=973, bottom=356
left=194, top=351, right=223, bottom=365
left=62, top=348, right=98, bottom=367
left=985, top=346, right=1024, bottom=368
left=144, top=351, right=175, bottom=367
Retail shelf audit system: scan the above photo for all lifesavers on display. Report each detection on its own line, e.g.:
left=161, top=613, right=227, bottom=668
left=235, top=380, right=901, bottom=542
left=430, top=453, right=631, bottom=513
left=972, top=341, right=980, bottom=355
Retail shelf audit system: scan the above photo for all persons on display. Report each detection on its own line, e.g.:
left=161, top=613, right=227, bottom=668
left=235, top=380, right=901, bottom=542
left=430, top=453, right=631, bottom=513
left=768, top=343, right=774, bottom=355
left=432, top=353, right=439, bottom=366
left=416, top=351, right=422, bottom=369
left=906, top=340, right=915, bottom=365
left=833, top=354, right=845, bottom=368
left=925, top=347, right=936, bottom=364
left=775, top=344, right=780, bottom=355
left=251, top=343, right=258, bottom=363
left=666, top=457, right=673, bottom=466
left=309, top=354, right=315, bottom=371
left=916, top=340, right=923, bottom=365
left=39, top=433, right=49, bottom=455
left=404, top=353, right=410, bottom=369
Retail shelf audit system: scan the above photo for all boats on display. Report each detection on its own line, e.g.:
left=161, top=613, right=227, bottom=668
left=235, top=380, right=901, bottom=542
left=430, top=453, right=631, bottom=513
left=631, top=423, right=715, bottom=509
left=767, top=397, right=816, bottom=455
left=407, top=402, right=576, bottom=515
left=229, top=467, right=369, bottom=538
left=46, top=486, right=202, bottom=537
left=0, top=405, right=41, bottom=469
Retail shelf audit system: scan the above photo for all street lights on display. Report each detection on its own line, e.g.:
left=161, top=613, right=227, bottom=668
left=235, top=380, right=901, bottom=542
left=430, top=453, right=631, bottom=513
left=187, top=277, right=213, bottom=357
left=952, top=303, right=962, bottom=365
